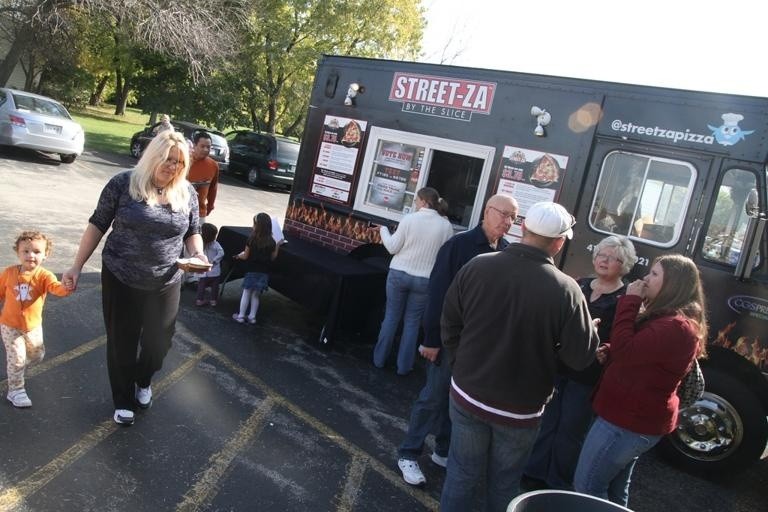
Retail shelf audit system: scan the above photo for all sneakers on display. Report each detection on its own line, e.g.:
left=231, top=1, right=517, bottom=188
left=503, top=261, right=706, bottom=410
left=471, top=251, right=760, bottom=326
left=196, top=299, right=207, bottom=307
left=134, top=383, right=155, bottom=410
left=113, top=408, right=136, bottom=426
left=5, top=387, right=33, bottom=408
left=209, top=299, right=218, bottom=307
left=429, top=453, right=447, bottom=466
left=396, top=457, right=426, bottom=487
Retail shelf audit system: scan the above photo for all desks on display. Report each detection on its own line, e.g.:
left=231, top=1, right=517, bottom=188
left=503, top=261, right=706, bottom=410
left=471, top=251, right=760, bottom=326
left=216, top=226, right=394, bottom=346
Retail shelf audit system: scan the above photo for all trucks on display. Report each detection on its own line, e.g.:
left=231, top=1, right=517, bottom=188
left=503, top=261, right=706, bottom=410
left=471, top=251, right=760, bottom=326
left=283, top=53, right=768, bottom=479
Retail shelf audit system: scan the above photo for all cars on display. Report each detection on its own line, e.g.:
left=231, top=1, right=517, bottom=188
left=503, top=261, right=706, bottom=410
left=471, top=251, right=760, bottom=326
left=0, top=88, right=85, bottom=163
left=130, top=121, right=230, bottom=176
left=225, top=130, right=301, bottom=191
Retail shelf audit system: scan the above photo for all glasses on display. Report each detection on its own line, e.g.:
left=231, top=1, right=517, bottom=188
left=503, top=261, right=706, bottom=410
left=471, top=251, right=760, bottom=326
left=488, top=206, right=517, bottom=221
left=597, top=253, right=623, bottom=264
left=558, top=214, right=577, bottom=235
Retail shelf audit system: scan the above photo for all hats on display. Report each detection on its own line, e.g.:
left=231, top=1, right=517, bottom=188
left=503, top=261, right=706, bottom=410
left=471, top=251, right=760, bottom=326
left=523, top=201, right=578, bottom=240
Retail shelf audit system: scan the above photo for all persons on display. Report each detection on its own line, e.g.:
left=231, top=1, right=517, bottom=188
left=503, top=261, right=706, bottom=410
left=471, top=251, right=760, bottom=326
left=1, top=229, right=74, bottom=408
left=574, top=256, right=708, bottom=507
left=185, top=131, right=219, bottom=231
left=368, top=188, right=452, bottom=377
left=437, top=202, right=600, bottom=512
left=231, top=213, right=276, bottom=324
left=194, top=223, right=224, bottom=307
left=153, top=115, right=174, bottom=133
left=61, top=129, right=212, bottom=426
left=524, top=234, right=639, bottom=489
left=397, top=194, right=518, bottom=487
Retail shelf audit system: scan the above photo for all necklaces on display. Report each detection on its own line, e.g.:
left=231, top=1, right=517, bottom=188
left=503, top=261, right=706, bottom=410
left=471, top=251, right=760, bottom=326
left=149, top=180, right=171, bottom=196
left=18, top=266, right=38, bottom=310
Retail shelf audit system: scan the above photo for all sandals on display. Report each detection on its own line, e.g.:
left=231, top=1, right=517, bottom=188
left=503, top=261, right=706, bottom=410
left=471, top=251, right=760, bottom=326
left=232, top=312, right=244, bottom=323
left=247, top=315, right=257, bottom=325
